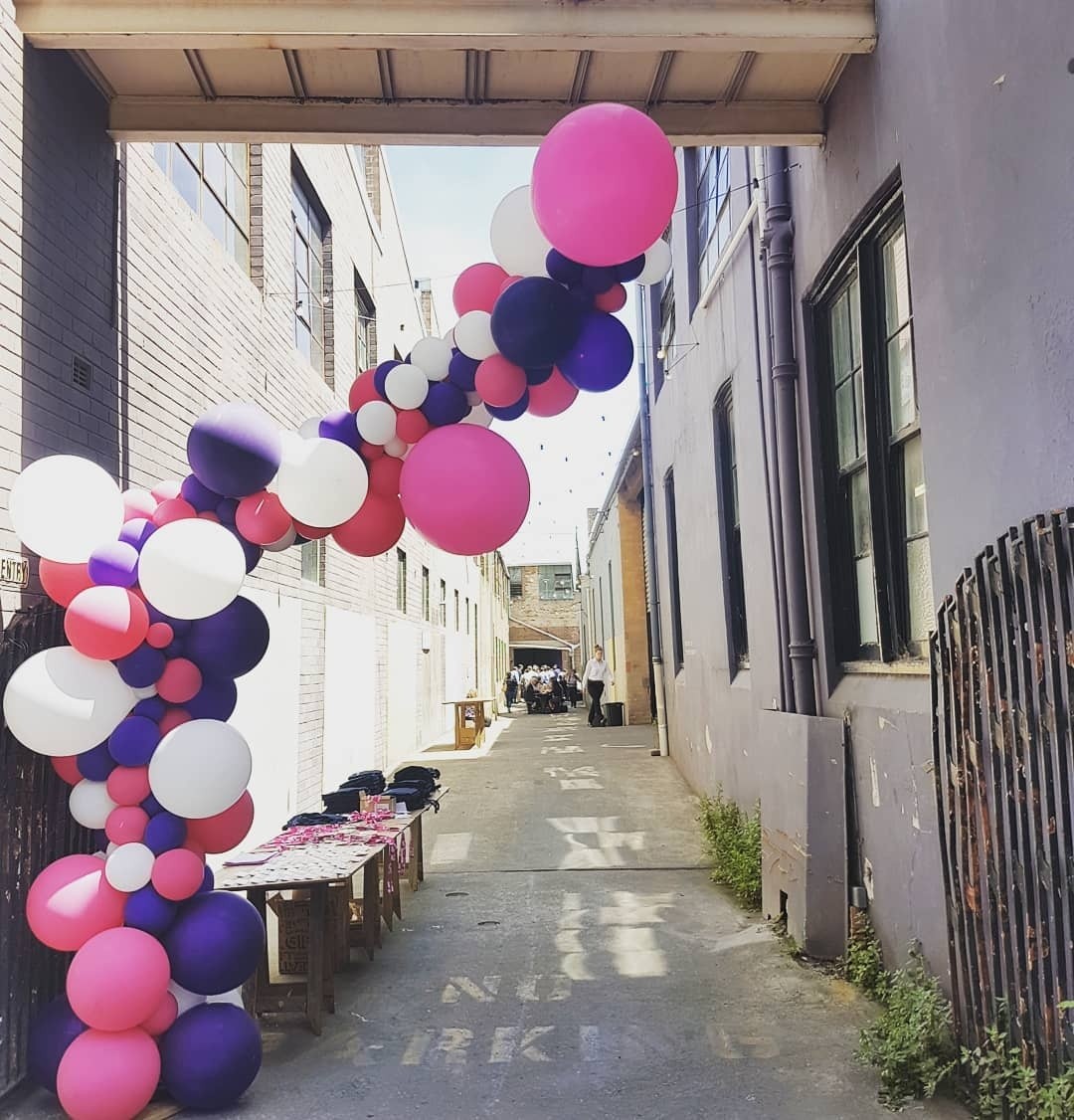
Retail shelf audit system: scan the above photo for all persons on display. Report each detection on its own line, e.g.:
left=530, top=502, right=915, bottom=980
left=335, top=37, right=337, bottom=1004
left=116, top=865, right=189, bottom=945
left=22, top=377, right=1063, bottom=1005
left=582, top=644, right=615, bottom=726
left=502, top=663, right=582, bottom=714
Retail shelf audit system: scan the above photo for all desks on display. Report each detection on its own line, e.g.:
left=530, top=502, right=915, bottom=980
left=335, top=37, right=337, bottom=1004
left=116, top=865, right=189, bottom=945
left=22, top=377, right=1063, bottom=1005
left=442, top=699, right=496, bottom=749
left=214, top=786, right=449, bottom=1037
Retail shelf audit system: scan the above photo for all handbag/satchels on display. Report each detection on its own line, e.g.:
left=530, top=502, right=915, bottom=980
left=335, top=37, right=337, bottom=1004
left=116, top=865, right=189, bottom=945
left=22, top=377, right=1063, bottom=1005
left=502, top=681, right=508, bottom=692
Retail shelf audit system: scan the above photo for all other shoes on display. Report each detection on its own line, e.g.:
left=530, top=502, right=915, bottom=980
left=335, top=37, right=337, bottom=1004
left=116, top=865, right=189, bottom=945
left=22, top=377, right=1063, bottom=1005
left=587, top=722, right=593, bottom=728
left=600, top=718, right=607, bottom=724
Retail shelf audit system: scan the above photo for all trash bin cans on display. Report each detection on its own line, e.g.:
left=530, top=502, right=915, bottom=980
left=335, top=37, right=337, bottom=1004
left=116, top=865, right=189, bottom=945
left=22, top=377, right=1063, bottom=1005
left=601, top=702, right=624, bottom=726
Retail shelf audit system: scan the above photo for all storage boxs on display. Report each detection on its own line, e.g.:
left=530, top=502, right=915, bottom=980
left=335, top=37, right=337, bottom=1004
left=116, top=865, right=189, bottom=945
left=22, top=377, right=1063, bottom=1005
left=351, top=898, right=382, bottom=946
left=360, top=796, right=396, bottom=812
left=267, top=885, right=351, bottom=973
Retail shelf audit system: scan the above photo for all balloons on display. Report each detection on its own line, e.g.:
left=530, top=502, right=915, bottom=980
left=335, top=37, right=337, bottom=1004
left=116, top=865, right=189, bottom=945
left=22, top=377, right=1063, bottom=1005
left=3, top=105, right=293, bottom=1120
left=277, top=105, right=680, bottom=555
